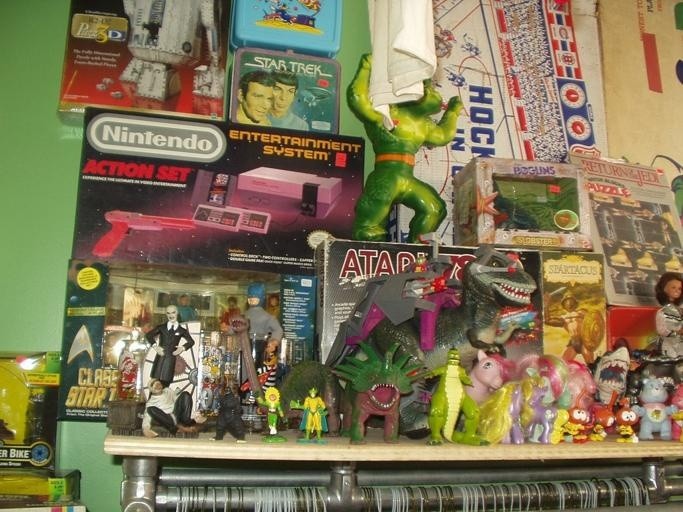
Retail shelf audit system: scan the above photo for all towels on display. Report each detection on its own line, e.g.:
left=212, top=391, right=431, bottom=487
left=362, top=0, right=438, bottom=130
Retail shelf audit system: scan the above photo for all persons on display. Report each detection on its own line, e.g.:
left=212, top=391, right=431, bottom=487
left=138, top=294, right=283, bottom=439
left=290, top=385, right=328, bottom=442
left=269, top=71, right=311, bottom=131
left=236, top=70, right=274, bottom=127
left=656, top=272, right=683, bottom=357
